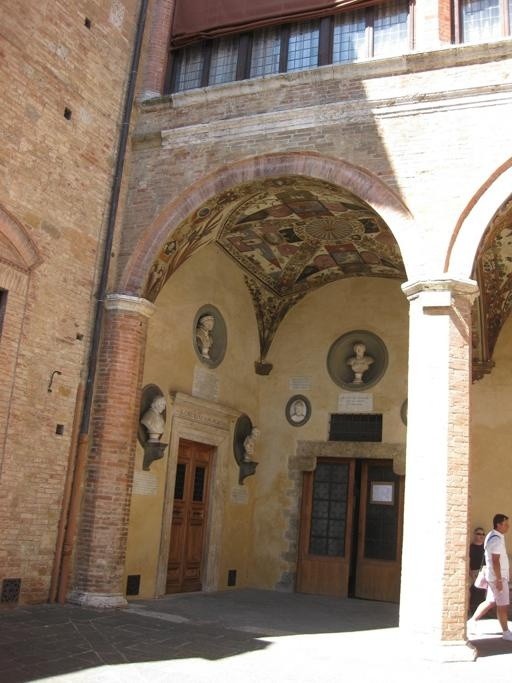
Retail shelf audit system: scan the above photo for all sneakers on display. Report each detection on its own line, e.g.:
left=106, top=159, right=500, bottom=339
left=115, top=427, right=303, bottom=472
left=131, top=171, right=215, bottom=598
left=502, top=631, right=512, bottom=642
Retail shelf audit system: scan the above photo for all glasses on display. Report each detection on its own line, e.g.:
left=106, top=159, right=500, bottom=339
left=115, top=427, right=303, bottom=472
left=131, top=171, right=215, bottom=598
left=475, top=533, right=486, bottom=536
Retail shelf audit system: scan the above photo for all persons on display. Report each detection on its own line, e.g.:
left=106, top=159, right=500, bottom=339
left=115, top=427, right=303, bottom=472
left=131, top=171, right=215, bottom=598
left=141, top=393, right=167, bottom=432
left=197, top=313, right=216, bottom=349
left=244, top=426, right=261, bottom=455
left=467, top=513, right=511, bottom=641
left=291, top=400, right=305, bottom=422
left=468, top=526, right=485, bottom=618
left=345, top=339, right=375, bottom=372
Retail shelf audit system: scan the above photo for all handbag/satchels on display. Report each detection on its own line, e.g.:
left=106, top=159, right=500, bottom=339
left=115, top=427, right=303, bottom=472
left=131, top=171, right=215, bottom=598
left=474, top=566, right=489, bottom=590
